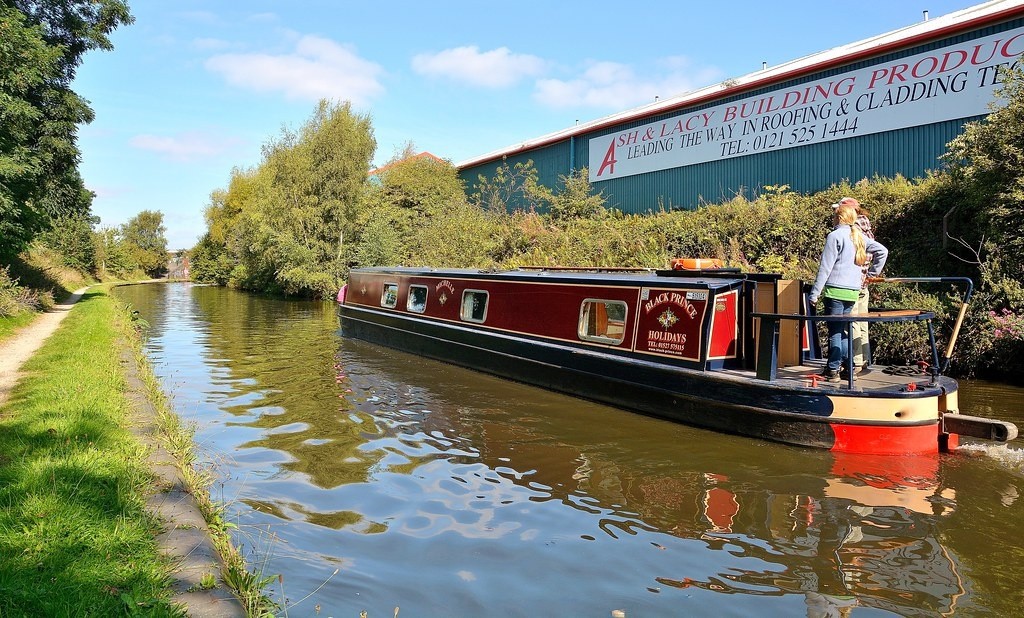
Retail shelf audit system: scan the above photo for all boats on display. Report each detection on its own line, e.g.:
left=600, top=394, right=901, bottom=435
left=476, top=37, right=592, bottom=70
left=337, top=257, right=1018, bottom=456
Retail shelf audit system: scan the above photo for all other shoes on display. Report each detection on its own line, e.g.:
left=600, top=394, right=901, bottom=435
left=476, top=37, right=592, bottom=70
left=839, top=371, right=857, bottom=381
left=816, top=368, right=840, bottom=383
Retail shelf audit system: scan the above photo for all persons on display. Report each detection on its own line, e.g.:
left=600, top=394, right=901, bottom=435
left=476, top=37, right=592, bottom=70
left=831, top=197, right=873, bottom=375
left=809, top=204, right=888, bottom=382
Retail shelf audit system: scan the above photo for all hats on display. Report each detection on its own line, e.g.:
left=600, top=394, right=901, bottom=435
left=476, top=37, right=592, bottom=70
left=831, top=197, right=860, bottom=214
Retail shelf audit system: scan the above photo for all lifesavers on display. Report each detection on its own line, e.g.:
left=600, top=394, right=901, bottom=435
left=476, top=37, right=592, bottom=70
left=668, top=256, right=726, bottom=271
